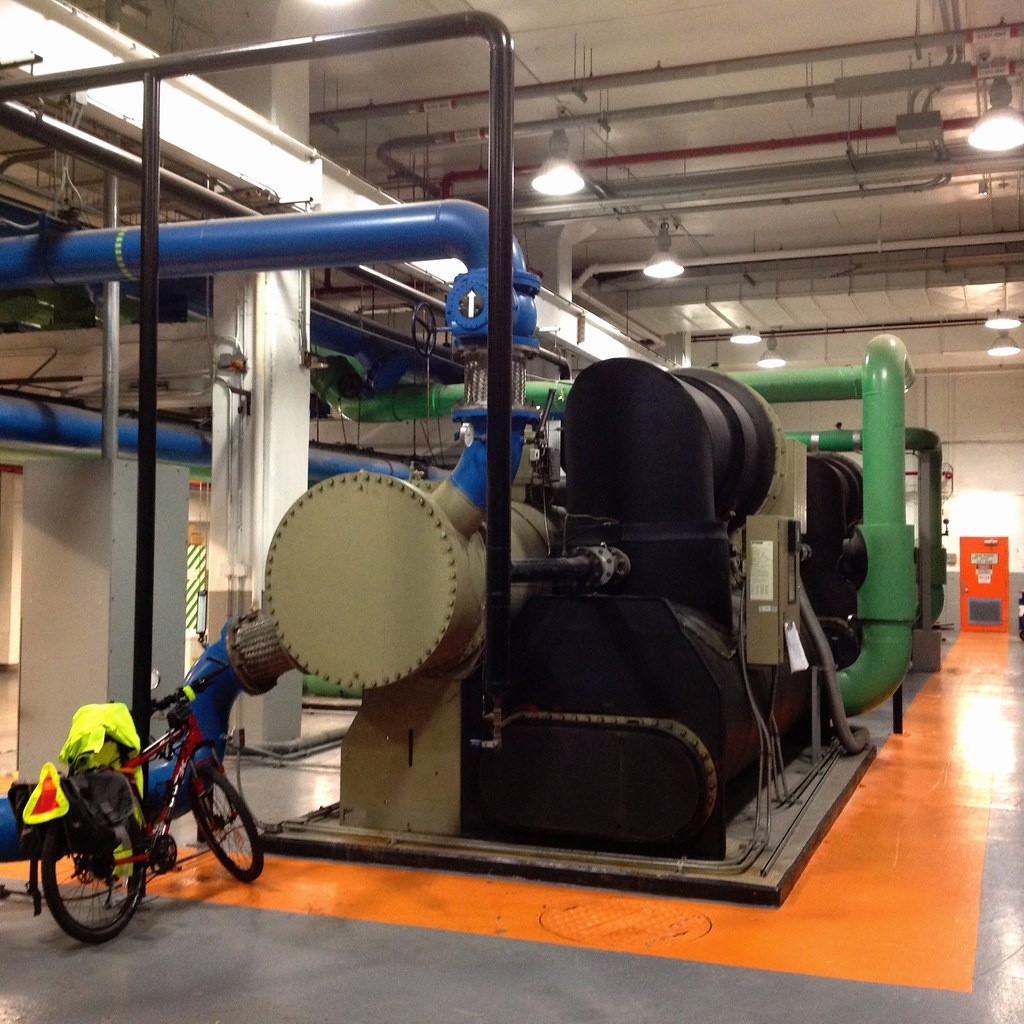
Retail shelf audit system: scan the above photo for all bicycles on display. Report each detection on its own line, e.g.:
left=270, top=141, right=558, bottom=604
left=21, top=658, right=264, bottom=942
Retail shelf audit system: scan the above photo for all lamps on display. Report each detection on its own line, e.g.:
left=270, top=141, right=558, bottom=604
left=730, top=325, right=762, bottom=344
left=532, top=129, right=585, bottom=196
left=985, top=311, right=1021, bottom=329
left=988, top=329, right=1020, bottom=356
left=642, top=222, right=684, bottom=278
left=978, top=173, right=988, bottom=196
left=967, top=76, right=1024, bottom=151
left=757, top=330, right=788, bottom=368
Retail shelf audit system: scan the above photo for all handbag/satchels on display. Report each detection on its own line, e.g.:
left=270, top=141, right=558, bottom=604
left=8, top=770, right=136, bottom=848
